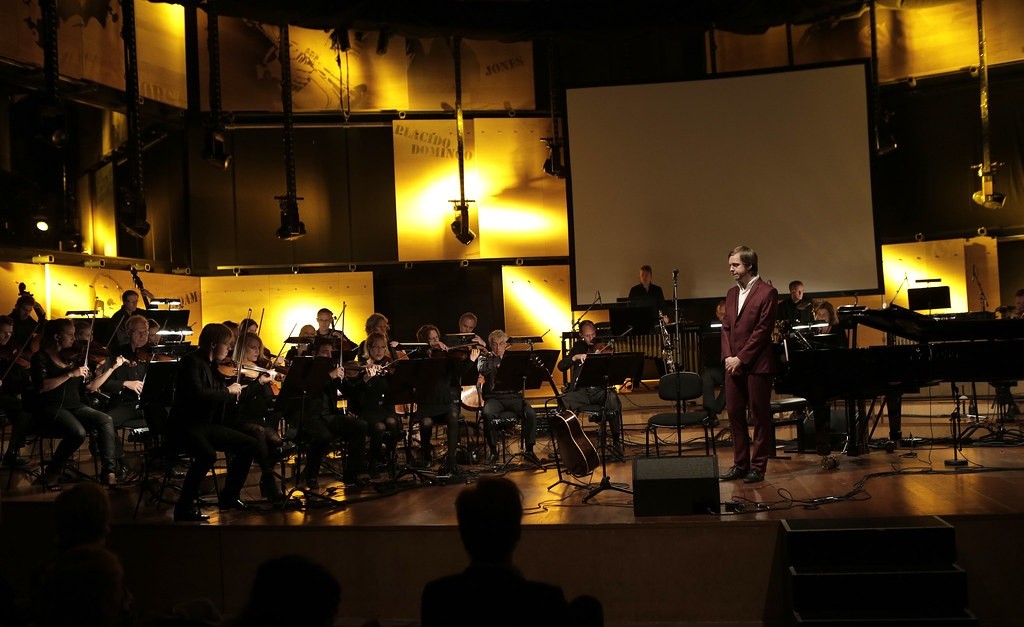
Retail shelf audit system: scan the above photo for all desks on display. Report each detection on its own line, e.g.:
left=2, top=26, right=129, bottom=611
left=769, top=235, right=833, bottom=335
left=563, top=325, right=702, bottom=386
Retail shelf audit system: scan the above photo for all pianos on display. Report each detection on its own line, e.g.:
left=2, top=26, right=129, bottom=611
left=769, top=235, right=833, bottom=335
left=775, top=302, right=1024, bottom=470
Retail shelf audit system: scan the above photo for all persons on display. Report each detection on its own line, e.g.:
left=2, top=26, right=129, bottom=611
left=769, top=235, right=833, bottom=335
left=996, top=290, right=1024, bottom=417
left=790, top=299, right=849, bottom=420
left=477, top=329, right=541, bottom=465
left=317, top=308, right=358, bottom=361
left=0, top=294, right=118, bottom=493
left=223, top=321, right=239, bottom=358
left=701, top=300, right=726, bottom=428
left=627, top=265, right=669, bottom=392
left=420, top=475, right=603, bottom=627
left=31, top=482, right=343, bottom=627
left=167, top=323, right=257, bottom=520
left=274, top=339, right=369, bottom=489
left=776, top=281, right=813, bottom=322
left=458, top=312, right=491, bottom=350
left=348, top=313, right=460, bottom=468
left=549, top=320, right=624, bottom=458
left=108, top=291, right=151, bottom=343
left=99, top=315, right=161, bottom=482
left=719, top=244, right=778, bottom=482
left=232, top=318, right=289, bottom=504
left=284, top=325, right=316, bottom=365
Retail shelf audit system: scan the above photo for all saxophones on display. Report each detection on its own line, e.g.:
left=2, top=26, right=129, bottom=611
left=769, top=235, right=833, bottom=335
left=659, top=309, right=675, bottom=374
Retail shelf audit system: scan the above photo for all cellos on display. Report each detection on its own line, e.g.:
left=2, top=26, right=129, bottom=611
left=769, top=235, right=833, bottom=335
left=130, top=266, right=150, bottom=310
left=385, top=332, right=418, bottom=446
left=460, top=372, right=486, bottom=414
left=331, top=313, right=350, bottom=351
left=18, top=282, right=49, bottom=353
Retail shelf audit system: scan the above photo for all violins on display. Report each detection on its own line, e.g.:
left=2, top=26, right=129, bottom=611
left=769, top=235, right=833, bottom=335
left=380, top=356, right=399, bottom=374
left=263, top=348, right=293, bottom=367
left=255, top=358, right=289, bottom=374
left=431, top=346, right=493, bottom=361
left=212, top=356, right=286, bottom=382
left=136, top=341, right=182, bottom=362
left=338, top=361, right=388, bottom=377
left=593, top=343, right=614, bottom=353
left=59, top=347, right=137, bottom=367
left=0, top=340, right=37, bottom=368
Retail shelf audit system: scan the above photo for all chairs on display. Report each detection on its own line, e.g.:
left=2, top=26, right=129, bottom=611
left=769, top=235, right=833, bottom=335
left=0, top=371, right=808, bottom=524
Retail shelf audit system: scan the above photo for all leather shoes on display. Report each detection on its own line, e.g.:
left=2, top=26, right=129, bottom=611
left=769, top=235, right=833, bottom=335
left=720, top=467, right=745, bottom=480
left=744, top=470, right=760, bottom=484
left=3, top=448, right=559, bottom=524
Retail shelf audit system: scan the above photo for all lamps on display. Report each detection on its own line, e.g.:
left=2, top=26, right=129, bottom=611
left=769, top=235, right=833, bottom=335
left=201, top=0, right=235, bottom=172
left=451, top=37, right=475, bottom=245
left=970, top=0, right=1008, bottom=211
left=792, top=320, right=829, bottom=330
left=274, top=20, right=307, bottom=242
left=867, top=0, right=898, bottom=156
left=540, top=0, right=567, bottom=179
left=119, top=0, right=152, bottom=240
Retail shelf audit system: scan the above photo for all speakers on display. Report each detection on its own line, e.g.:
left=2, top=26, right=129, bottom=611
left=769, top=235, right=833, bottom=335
left=632, top=454, right=721, bottom=518
left=802, top=407, right=869, bottom=449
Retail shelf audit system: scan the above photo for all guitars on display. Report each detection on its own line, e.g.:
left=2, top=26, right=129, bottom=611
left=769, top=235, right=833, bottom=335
left=529, top=353, right=601, bottom=476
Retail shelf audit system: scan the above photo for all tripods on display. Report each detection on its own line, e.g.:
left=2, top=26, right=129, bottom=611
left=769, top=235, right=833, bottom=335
left=956, top=382, right=996, bottom=442
left=272, top=350, right=645, bottom=512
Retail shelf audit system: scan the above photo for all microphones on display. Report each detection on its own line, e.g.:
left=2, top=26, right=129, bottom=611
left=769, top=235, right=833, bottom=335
left=598, top=291, right=603, bottom=307
left=972, top=264, right=976, bottom=281
left=673, top=268, right=679, bottom=280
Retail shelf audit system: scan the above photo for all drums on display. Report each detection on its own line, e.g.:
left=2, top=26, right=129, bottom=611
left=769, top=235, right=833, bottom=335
left=149, top=340, right=197, bottom=357
left=444, top=332, right=475, bottom=345
